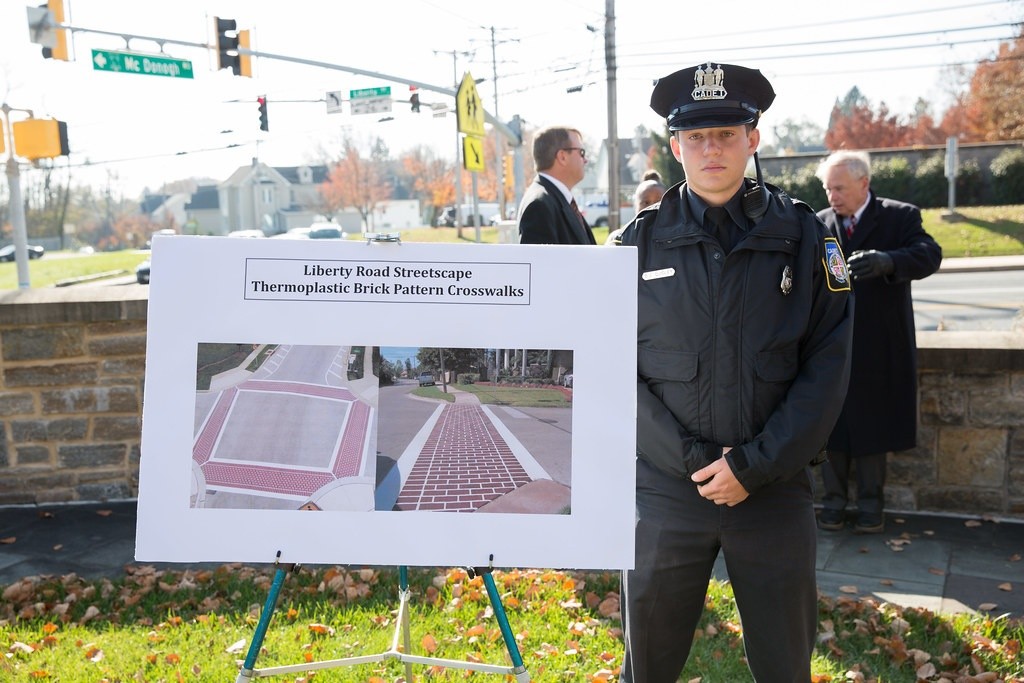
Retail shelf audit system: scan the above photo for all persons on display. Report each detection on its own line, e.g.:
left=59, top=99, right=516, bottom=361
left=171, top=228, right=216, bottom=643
left=603, top=61, right=854, bottom=683
left=633, top=169, right=668, bottom=216
left=816, top=148, right=942, bottom=533
left=516, top=127, right=597, bottom=244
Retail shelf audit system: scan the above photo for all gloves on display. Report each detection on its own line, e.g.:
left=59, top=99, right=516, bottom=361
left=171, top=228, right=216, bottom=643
left=847, top=249, right=894, bottom=280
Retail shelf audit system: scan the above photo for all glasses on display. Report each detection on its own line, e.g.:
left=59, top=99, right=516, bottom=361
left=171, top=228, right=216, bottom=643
left=559, top=146, right=585, bottom=157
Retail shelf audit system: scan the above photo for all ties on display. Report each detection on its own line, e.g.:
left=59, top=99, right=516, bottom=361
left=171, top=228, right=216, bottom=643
left=568, top=196, right=591, bottom=241
left=843, top=215, right=856, bottom=240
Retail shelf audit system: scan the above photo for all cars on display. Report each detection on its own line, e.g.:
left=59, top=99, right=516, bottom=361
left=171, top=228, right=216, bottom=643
left=0, top=244, right=44, bottom=264
left=136, top=258, right=150, bottom=284
left=308, top=223, right=342, bottom=239
left=581, top=201, right=611, bottom=227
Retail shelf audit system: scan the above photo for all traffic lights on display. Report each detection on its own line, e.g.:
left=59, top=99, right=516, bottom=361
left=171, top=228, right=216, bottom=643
left=410, top=94, right=419, bottom=113
left=258, top=97, right=268, bottom=131
left=213, top=16, right=238, bottom=70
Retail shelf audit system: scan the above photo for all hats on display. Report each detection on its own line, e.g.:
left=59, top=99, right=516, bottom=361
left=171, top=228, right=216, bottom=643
left=651, top=61, right=777, bottom=131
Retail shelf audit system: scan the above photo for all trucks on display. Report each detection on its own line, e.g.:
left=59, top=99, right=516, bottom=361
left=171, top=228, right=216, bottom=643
left=436, top=203, right=502, bottom=228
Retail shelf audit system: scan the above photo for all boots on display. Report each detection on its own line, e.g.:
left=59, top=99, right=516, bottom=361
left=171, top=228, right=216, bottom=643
left=818, top=448, right=850, bottom=531
left=849, top=453, right=885, bottom=533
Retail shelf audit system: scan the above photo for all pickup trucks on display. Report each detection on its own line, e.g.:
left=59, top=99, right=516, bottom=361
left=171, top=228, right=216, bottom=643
left=418, top=372, right=435, bottom=386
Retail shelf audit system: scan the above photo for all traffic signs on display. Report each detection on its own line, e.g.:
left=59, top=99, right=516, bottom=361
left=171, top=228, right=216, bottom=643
left=92, top=49, right=194, bottom=79
